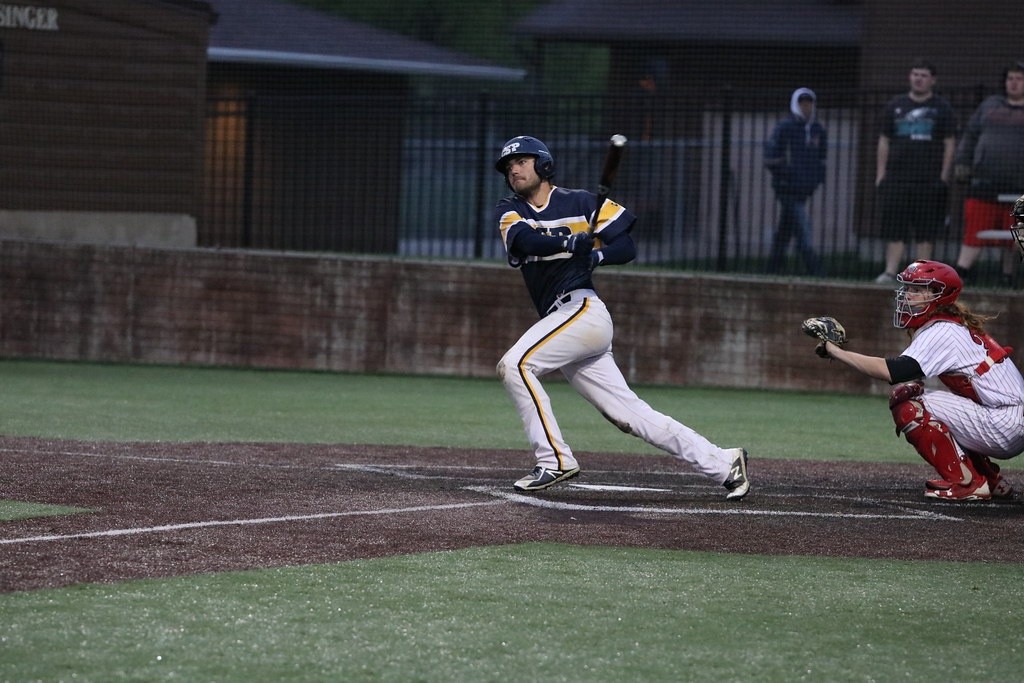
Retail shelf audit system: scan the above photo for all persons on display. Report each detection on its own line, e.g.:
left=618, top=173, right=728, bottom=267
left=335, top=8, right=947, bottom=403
left=493, top=134, right=752, bottom=501
left=763, top=86, right=830, bottom=279
left=951, top=60, right=1023, bottom=286
left=871, top=59, right=960, bottom=284
left=801, top=258, right=1024, bottom=501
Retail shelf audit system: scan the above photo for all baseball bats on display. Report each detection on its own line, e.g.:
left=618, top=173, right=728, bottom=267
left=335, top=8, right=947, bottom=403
left=586, top=133, right=628, bottom=238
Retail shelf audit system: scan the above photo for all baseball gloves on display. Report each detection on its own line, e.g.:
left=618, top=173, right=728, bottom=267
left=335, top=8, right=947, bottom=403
left=803, top=316, right=848, bottom=363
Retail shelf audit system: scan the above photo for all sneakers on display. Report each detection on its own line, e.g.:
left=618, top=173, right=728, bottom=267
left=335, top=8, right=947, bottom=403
left=514, top=467, right=579, bottom=491
left=723, top=448, right=750, bottom=501
left=923, top=463, right=1014, bottom=503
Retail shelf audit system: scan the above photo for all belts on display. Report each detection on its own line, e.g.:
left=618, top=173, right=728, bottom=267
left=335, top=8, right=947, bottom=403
left=540, top=293, right=571, bottom=319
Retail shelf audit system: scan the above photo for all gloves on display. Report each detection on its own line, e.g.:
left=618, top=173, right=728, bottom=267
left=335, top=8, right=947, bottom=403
left=569, top=232, right=599, bottom=268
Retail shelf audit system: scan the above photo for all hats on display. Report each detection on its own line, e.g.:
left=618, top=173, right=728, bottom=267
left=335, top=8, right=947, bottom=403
left=798, top=93, right=814, bottom=103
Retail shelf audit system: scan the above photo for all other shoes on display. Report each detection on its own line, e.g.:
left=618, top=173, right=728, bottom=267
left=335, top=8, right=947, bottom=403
left=994, top=277, right=1016, bottom=289
left=875, top=272, right=897, bottom=282
left=953, top=266, right=970, bottom=283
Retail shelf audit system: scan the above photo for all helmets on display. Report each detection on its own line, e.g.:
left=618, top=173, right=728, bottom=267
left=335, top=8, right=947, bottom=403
left=892, top=259, right=964, bottom=329
left=495, top=136, right=556, bottom=194
